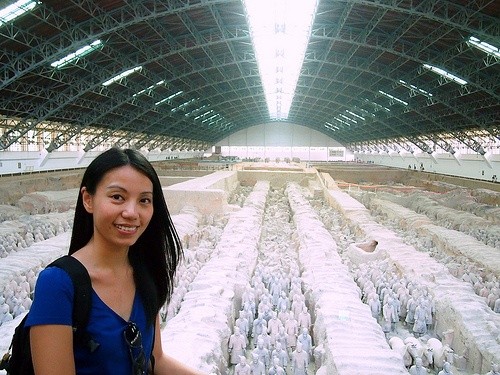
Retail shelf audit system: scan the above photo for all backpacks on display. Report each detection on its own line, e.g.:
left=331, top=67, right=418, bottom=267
left=0, top=255, right=155, bottom=375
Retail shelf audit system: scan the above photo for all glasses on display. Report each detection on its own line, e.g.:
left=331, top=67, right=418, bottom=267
left=124, top=321, right=146, bottom=375
